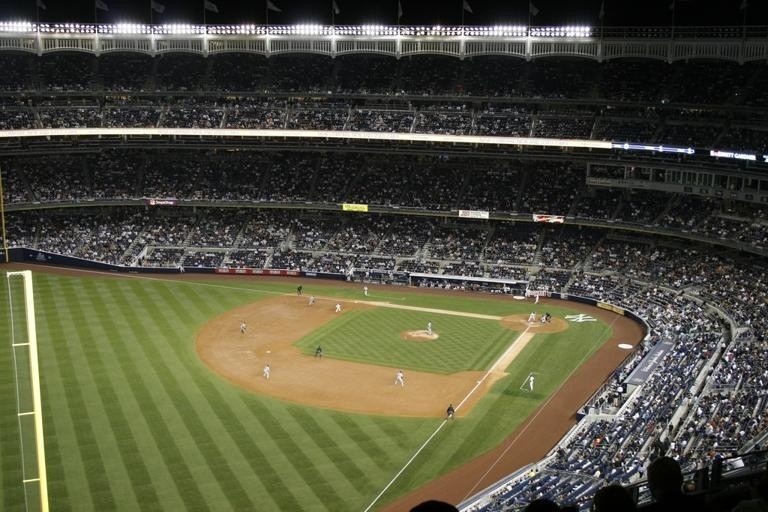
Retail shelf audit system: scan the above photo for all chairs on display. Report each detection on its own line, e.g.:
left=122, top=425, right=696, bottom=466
left=119, top=231, right=598, bottom=303
left=465, top=229, right=768, bottom=510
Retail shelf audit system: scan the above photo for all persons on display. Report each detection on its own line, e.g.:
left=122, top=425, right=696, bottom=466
left=395, top=369, right=403, bottom=388
left=309, top=296, right=315, bottom=305
left=240, top=320, right=246, bottom=335
left=460, top=292, right=767, bottom=512
left=335, top=302, right=342, bottom=311
left=445, top=402, right=455, bottom=421
left=315, top=346, right=321, bottom=359
left=427, top=320, right=433, bottom=337
left=0, top=50, right=768, bottom=295
left=262, top=363, right=271, bottom=380
